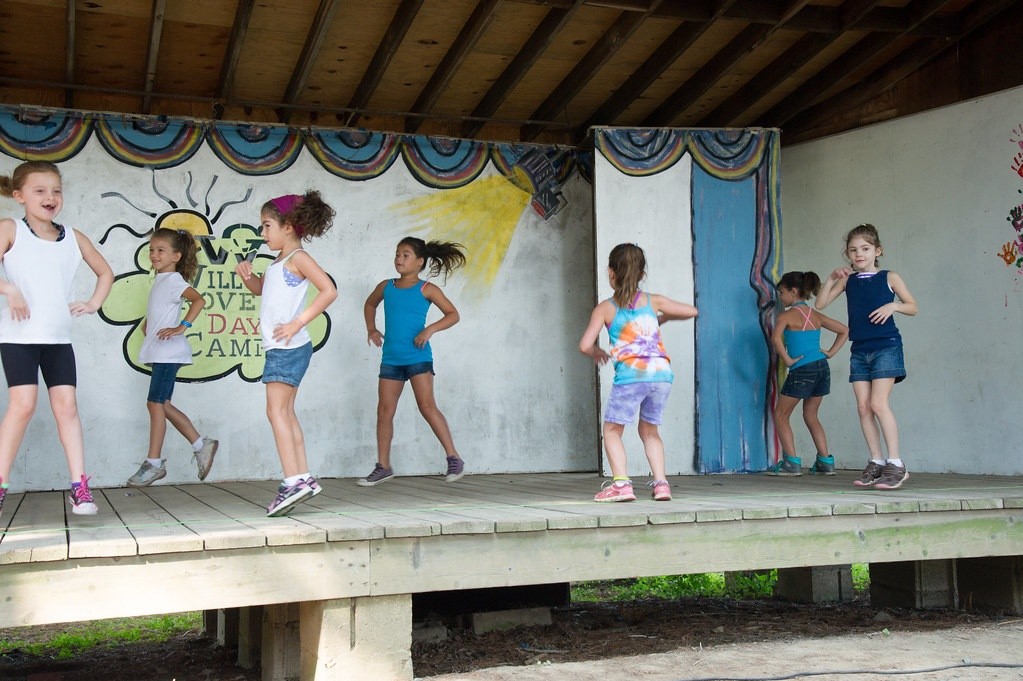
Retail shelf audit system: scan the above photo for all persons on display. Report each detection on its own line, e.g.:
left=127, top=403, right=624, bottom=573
left=0, top=160, right=115, bottom=515
left=578, top=243, right=698, bottom=503
left=357, top=236, right=466, bottom=486
left=765, top=271, right=849, bottom=477
left=235, top=190, right=339, bottom=518
left=815, top=223, right=917, bottom=488
left=126, top=227, right=219, bottom=487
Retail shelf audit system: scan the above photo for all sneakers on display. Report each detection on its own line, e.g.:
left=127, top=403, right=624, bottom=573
left=127, top=459, right=167, bottom=489
left=445, top=455, right=466, bottom=483
left=67, top=474, right=99, bottom=516
left=190, top=437, right=218, bottom=481
left=265, top=476, right=324, bottom=518
left=593, top=480, right=636, bottom=503
left=807, top=454, right=837, bottom=476
left=356, top=462, right=395, bottom=488
left=851, top=460, right=888, bottom=487
left=760, top=454, right=804, bottom=477
left=0, top=475, right=8, bottom=517
left=873, top=459, right=910, bottom=490
left=646, top=480, right=672, bottom=501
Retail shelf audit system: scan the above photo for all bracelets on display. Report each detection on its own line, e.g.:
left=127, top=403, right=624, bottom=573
left=296, top=317, right=306, bottom=326
left=181, top=320, right=192, bottom=327
